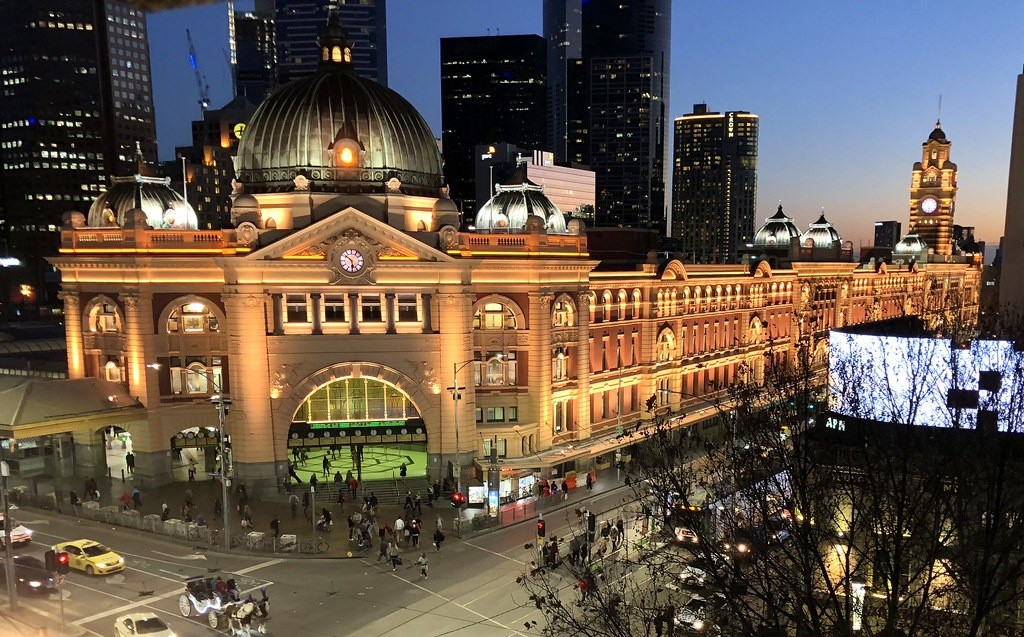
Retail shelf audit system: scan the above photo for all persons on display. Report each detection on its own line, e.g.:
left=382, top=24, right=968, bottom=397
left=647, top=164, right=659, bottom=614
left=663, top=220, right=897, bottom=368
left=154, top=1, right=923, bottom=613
left=160, top=477, right=281, bottom=538
left=585, top=473, right=594, bottom=492
left=400, top=463, right=407, bottom=485
left=578, top=576, right=588, bottom=602
left=188, top=458, right=196, bottom=476
left=282, top=444, right=380, bottom=554
left=82, top=475, right=100, bottom=501
left=71, top=489, right=79, bottom=506
left=376, top=537, right=399, bottom=571
left=443, top=478, right=449, bottom=499
left=193, top=576, right=241, bottom=602
left=417, top=552, right=429, bottom=579
left=532, top=480, right=568, bottom=497
left=126, top=452, right=135, bottom=474
left=379, top=514, right=424, bottom=548
left=427, top=479, right=442, bottom=507
left=120, top=484, right=144, bottom=511
left=404, top=488, right=422, bottom=521
left=432, top=513, right=445, bottom=552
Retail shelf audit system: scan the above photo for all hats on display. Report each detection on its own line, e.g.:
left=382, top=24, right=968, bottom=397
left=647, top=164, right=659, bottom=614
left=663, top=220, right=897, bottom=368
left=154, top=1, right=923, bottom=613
left=412, top=520, right=416, bottom=523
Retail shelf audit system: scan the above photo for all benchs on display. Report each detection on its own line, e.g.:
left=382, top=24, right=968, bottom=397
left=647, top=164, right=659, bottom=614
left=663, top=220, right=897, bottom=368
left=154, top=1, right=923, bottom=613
left=212, top=592, right=234, bottom=598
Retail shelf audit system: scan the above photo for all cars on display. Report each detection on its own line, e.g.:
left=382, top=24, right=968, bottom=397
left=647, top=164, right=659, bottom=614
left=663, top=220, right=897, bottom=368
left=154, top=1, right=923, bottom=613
left=673, top=590, right=729, bottom=637
left=677, top=552, right=735, bottom=587
left=113, top=611, right=179, bottom=637
left=0, top=512, right=33, bottom=551
left=742, top=424, right=792, bottom=470
left=675, top=526, right=699, bottom=546
left=0, top=554, right=57, bottom=595
left=718, top=506, right=792, bottom=565
left=51, top=538, right=127, bottom=577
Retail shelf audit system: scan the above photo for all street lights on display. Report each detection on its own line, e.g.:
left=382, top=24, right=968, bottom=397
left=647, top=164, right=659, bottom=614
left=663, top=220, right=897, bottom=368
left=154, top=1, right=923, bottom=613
left=145, top=363, right=233, bottom=550
left=453, top=358, right=483, bottom=539
left=617, top=363, right=639, bottom=482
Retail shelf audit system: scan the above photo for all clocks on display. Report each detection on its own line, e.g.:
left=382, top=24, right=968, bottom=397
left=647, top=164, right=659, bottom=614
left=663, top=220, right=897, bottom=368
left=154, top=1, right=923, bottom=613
left=339, top=249, right=364, bottom=274
left=921, top=198, right=938, bottom=214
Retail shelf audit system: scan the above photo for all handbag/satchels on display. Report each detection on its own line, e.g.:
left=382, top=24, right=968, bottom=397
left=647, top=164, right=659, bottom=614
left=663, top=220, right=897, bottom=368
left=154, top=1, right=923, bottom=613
left=242, top=519, right=248, bottom=528
left=440, top=533, right=444, bottom=541
left=396, top=558, right=402, bottom=565
left=433, top=543, right=436, bottom=547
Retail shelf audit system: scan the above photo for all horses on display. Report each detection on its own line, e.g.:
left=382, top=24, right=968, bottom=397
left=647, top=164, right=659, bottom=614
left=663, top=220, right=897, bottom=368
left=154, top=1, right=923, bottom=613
left=222, top=595, right=271, bottom=637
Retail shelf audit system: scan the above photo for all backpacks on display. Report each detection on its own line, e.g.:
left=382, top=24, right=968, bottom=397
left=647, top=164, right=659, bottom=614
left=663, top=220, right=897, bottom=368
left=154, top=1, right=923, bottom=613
left=291, top=496, right=297, bottom=505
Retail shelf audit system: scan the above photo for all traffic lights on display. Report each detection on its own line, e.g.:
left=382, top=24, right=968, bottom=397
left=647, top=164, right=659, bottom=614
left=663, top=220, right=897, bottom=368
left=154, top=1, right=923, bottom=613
left=537, top=519, right=546, bottom=537
left=450, top=493, right=466, bottom=508
left=56, top=552, right=70, bottom=575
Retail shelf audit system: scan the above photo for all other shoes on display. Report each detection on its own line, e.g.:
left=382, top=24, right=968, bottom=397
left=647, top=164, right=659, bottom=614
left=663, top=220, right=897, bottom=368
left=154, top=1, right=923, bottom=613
left=349, top=539, right=354, bottom=540
left=393, top=569, right=395, bottom=571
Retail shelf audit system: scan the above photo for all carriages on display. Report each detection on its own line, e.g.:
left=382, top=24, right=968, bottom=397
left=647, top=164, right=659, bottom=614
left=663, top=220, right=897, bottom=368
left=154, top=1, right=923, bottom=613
left=179, top=576, right=273, bottom=637
